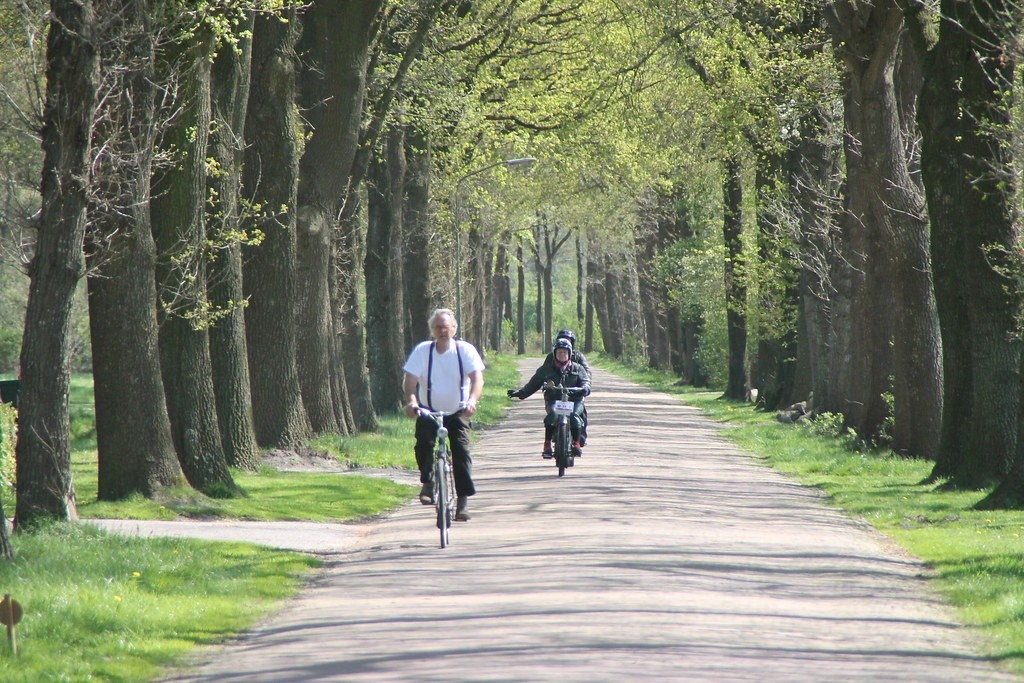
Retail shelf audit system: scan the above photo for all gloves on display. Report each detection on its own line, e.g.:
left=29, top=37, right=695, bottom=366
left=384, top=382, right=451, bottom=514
left=581, top=386, right=588, bottom=396
left=507, top=389, right=521, bottom=399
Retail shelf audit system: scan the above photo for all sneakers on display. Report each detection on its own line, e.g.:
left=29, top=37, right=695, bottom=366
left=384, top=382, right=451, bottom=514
left=543, top=440, right=552, bottom=453
left=573, top=441, right=582, bottom=450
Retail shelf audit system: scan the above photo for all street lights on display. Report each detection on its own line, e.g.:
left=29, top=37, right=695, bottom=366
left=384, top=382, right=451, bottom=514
left=454, top=158, right=535, bottom=340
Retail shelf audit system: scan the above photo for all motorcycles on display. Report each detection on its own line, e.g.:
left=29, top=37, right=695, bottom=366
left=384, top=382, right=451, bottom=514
left=542, top=381, right=588, bottom=476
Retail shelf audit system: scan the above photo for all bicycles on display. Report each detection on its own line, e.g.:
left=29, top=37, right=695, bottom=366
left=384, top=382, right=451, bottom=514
left=411, top=402, right=469, bottom=549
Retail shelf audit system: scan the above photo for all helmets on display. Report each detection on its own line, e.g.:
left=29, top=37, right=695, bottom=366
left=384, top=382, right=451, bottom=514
left=552, top=339, right=572, bottom=361
left=557, top=330, right=575, bottom=349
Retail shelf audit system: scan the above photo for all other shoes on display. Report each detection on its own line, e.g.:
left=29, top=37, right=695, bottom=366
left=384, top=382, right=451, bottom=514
left=455, top=507, right=471, bottom=519
left=419, top=482, right=435, bottom=504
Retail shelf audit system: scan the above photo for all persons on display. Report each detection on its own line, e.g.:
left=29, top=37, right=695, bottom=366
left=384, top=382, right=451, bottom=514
left=536, top=331, right=593, bottom=448
left=507, top=338, right=590, bottom=459
left=403, top=310, right=486, bottom=521
